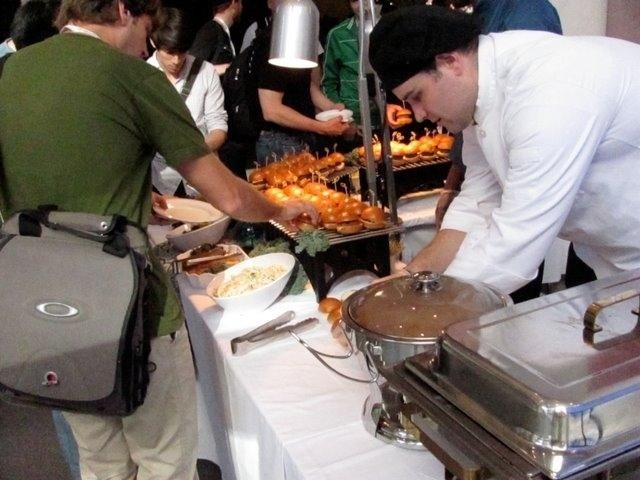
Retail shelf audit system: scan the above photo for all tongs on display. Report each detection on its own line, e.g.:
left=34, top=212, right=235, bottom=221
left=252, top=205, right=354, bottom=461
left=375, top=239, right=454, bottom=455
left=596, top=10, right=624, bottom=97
left=230, top=310, right=320, bottom=358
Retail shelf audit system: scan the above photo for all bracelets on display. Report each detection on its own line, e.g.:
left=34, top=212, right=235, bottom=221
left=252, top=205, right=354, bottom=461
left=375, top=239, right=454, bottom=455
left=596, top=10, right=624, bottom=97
left=441, top=189, right=457, bottom=197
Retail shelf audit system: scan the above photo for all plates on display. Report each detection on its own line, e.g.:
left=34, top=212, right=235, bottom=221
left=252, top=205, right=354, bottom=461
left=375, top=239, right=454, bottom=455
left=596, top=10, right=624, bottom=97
left=151, top=195, right=225, bottom=225
left=314, top=109, right=353, bottom=122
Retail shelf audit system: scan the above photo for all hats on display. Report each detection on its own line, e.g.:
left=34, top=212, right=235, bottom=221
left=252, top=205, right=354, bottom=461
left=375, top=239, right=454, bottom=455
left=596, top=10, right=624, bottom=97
left=367, top=6, right=482, bottom=95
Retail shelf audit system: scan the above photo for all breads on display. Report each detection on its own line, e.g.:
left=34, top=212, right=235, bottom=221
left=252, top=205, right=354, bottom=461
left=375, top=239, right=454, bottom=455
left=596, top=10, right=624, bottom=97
left=316, top=297, right=343, bottom=324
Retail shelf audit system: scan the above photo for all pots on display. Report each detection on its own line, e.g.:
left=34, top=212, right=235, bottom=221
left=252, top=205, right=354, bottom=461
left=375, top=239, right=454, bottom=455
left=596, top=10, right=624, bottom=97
left=290, top=272, right=514, bottom=453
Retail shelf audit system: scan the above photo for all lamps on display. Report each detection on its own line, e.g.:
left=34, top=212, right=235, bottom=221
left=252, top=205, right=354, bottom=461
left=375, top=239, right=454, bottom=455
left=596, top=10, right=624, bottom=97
left=266, top=1, right=319, bottom=70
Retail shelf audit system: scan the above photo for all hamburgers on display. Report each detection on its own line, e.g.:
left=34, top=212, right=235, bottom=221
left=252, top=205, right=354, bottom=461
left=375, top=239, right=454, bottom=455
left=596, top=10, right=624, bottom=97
left=249, top=134, right=456, bottom=236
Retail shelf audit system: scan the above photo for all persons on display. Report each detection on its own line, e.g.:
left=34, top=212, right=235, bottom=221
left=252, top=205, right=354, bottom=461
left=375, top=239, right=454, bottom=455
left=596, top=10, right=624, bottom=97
left=335, top=6, right=640, bottom=353
left=0, top=0, right=62, bottom=57
left=1, top=2, right=322, bottom=480
left=433, top=0, right=563, bottom=305
left=144, top=0, right=385, bottom=199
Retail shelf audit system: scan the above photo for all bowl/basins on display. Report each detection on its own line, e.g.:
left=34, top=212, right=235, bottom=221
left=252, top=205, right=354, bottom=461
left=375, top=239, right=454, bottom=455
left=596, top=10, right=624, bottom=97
left=164, top=214, right=231, bottom=252
left=206, top=252, right=295, bottom=313
left=181, top=242, right=250, bottom=288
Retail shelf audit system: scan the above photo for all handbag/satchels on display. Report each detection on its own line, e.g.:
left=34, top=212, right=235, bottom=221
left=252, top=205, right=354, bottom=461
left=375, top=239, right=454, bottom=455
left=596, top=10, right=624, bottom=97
left=0, top=205, right=161, bottom=419
